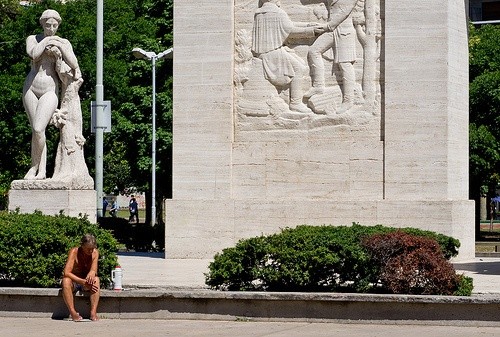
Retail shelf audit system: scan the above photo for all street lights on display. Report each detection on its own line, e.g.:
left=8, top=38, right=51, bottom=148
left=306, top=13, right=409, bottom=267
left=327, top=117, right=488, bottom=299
left=132, top=47, right=173, bottom=229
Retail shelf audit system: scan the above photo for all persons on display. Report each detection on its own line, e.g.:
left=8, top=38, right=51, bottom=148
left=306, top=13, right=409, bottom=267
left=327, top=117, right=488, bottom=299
left=109, top=197, right=117, bottom=217
left=128, top=195, right=139, bottom=223
left=300, top=0, right=365, bottom=114
left=21, top=10, right=82, bottom=180
left=103, top=197, right=108, bottom=217
left=251, top=0, right=324, bottom=113
left=61, top=233, right=100, bottom=321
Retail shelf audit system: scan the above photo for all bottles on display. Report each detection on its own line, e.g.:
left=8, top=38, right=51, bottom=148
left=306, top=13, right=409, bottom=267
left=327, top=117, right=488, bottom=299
left=111, top=266, right=122, bottom=290
